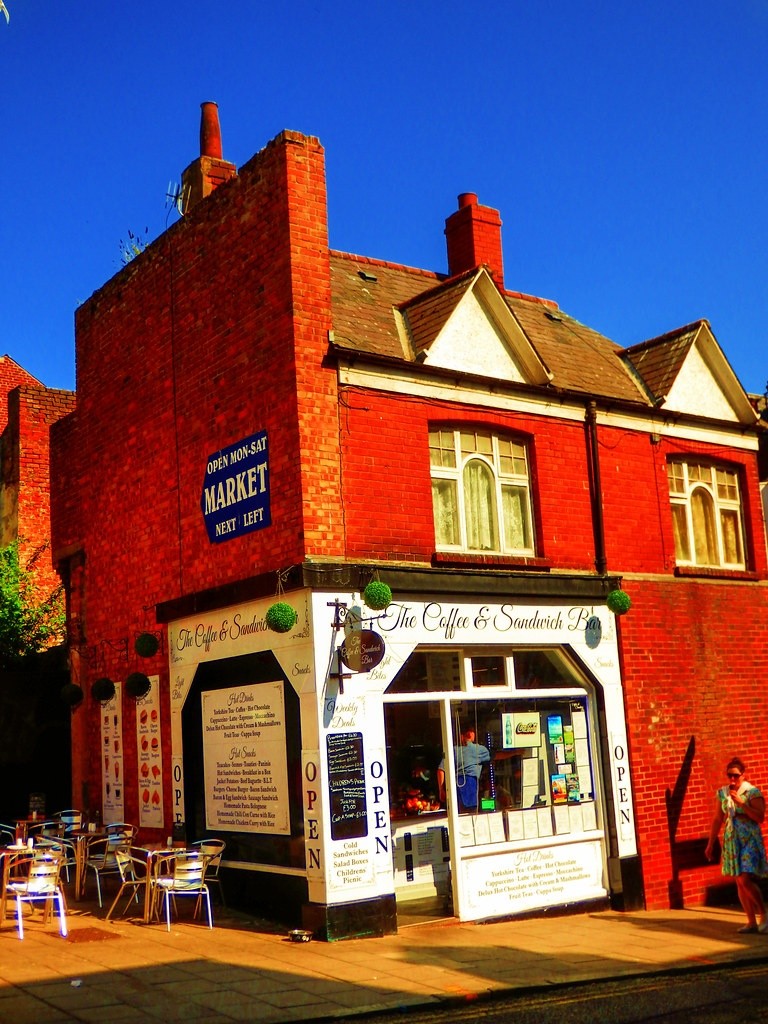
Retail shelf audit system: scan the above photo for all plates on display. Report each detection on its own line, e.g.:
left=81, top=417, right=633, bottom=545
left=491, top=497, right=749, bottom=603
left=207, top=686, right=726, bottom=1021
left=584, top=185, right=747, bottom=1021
left=8, top=845, right=28, bottom=850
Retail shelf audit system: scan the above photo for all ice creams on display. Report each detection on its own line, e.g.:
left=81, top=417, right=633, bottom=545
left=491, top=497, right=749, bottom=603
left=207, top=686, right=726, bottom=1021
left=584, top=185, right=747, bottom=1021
left=729, top=782, right=735, bottom=798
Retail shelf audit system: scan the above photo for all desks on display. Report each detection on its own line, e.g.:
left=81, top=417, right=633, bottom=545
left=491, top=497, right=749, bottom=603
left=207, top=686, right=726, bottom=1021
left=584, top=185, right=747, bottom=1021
left=71, top=832, right=113, bottom=900
left=141, top=842, right=193, bottom=924
left=0, top=845, right=56, bottom=923
left=13, top=819, right=60, bottom=844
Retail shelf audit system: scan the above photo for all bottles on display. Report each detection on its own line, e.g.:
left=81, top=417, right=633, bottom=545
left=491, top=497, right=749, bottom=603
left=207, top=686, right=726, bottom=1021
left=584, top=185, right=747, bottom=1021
left=506, top=715, right=512, bottom=744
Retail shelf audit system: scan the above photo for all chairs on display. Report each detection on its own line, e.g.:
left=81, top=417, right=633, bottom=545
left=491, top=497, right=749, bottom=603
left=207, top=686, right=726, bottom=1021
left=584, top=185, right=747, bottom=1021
left=0, top=809, right=228, bottom=939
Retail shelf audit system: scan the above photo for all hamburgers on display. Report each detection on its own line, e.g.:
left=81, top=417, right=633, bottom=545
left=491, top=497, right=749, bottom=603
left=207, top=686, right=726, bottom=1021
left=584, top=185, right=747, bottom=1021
left=140, top=710, right=159, bottom=752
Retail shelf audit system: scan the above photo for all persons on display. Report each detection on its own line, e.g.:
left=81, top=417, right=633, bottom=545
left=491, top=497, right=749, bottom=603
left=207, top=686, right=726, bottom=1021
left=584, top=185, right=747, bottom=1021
left=436, top=726, right=491, bottom=814
left=704, top=756, right=768, bottom=935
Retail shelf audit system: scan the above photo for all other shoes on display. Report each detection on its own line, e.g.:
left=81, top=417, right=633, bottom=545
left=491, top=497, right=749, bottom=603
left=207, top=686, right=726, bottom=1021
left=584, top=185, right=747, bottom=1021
left=737, top=924, right=758, bottom=933
left=758, top=916, right=768, bottom=933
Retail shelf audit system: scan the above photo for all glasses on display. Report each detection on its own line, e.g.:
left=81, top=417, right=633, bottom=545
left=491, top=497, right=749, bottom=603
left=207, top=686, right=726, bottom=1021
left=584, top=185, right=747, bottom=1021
left=726, top=773, right=743, bottom=777
